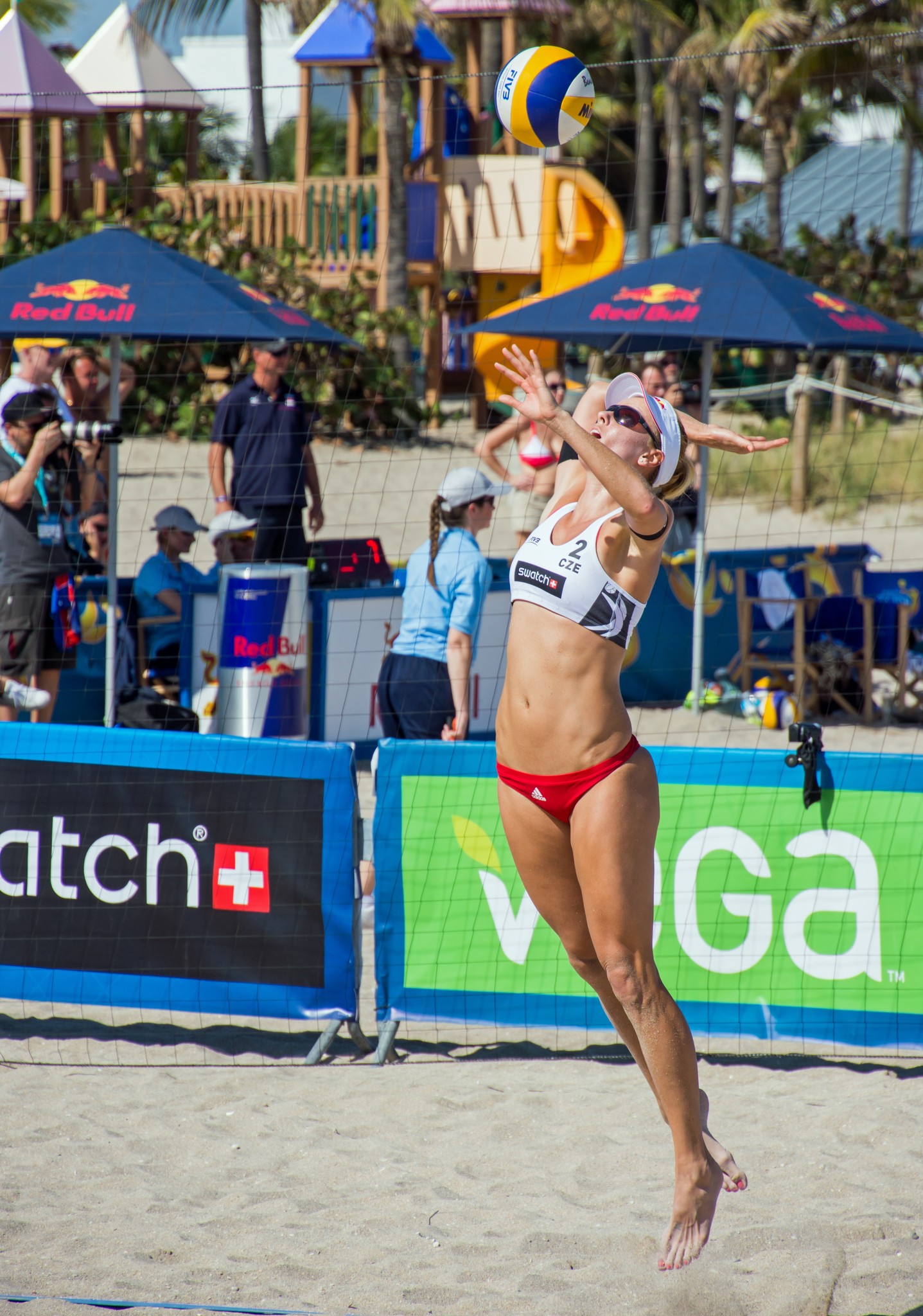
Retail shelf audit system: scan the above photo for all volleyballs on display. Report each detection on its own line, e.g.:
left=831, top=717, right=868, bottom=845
left=492, top=45, right=597, bottom=150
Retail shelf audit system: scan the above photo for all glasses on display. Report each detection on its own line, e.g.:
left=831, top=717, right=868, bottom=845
left=94, top=523, right=106, bottom=532
left=226, top=531, right=256, bottom=541
left=595, top=405, right=658, bottom=449
left=484, top=497, right=494, bottom=506
left=47, top=348, right=62, bottom=354
left=29, top=424, right=42, bottom=431
left=274, top=351, right=286, bottom=356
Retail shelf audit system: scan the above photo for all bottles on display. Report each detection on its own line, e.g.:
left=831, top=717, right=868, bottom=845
left=739, top=692, right=762, bottom=725
left=704, top=675, right=738, bottom=699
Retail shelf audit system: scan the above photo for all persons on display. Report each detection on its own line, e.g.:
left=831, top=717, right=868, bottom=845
left=471, top=358, right=705, bottom=552
left=132, top=505, right=210, bottom=674
left=377, top=467, right=512, bottom=741
left=209, top=336, right=325, bottom=565
left=494, top=340, right=790, bottom=1276
left=207, top=510, right=259, bottom=578
left=0, top=338, right=134, bottom=723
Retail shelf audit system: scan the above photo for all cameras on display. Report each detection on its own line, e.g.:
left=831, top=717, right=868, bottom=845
left=34, top=413, right=125, bottom=453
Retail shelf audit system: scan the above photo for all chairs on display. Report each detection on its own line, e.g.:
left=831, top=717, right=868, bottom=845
left=73, top=576, right=180, bottom=694
left=736, top=563, right=907, bottom=724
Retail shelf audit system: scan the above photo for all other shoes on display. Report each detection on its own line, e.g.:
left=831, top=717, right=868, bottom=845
left=0, top=680, right=52, bottom=710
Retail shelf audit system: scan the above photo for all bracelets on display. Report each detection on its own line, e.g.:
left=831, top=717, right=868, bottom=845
left=213, top=494, right=228, bottom=504
left=628, top=512, right=669, bottom=541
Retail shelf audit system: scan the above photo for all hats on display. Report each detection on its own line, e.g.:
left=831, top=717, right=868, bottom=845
left=439, top=467, right=511, bottom=512
left=252, top=341, right=290, bottom=351
left=150, top=505, right=208, bottom=533
left=13, top=338, right=70, bottom=353
left=604, top=373, right=680, bottom=488
left=208, top=511, right=257, bottom=546
left=1, top=392, right=57, bottom=426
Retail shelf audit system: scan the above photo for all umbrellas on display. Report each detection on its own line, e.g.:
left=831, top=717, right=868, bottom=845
left=0, top=224, right=367, bottom=729
left=454, top=237, right=923, bottom=717
